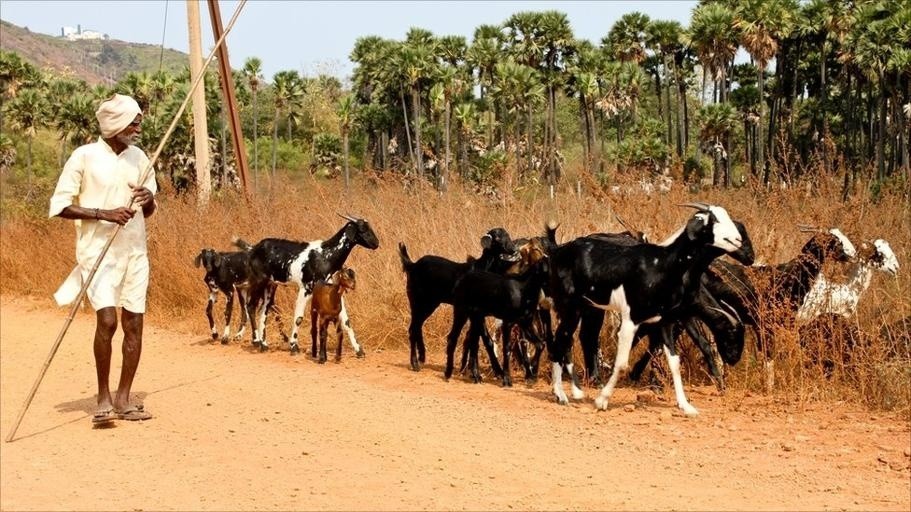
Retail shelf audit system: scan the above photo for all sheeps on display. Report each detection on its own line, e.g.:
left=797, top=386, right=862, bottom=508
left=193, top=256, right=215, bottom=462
left=399, top=201, right=900, bottom=417
left=194, top=209, right=379, bottom=365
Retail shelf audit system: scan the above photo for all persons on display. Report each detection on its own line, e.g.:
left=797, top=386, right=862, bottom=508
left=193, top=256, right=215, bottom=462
left=47, top=93, right=159, bottom=425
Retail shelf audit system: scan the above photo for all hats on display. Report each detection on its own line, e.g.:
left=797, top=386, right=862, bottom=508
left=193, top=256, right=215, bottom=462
left=95, top=93, right=143, bottom=139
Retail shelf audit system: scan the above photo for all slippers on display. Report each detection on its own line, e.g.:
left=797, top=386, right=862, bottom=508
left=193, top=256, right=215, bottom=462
left=92, top=405, right=153, bottom=423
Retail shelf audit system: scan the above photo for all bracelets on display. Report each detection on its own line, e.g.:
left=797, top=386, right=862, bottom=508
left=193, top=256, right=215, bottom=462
left=95, top=208, right=99, bottom=221
